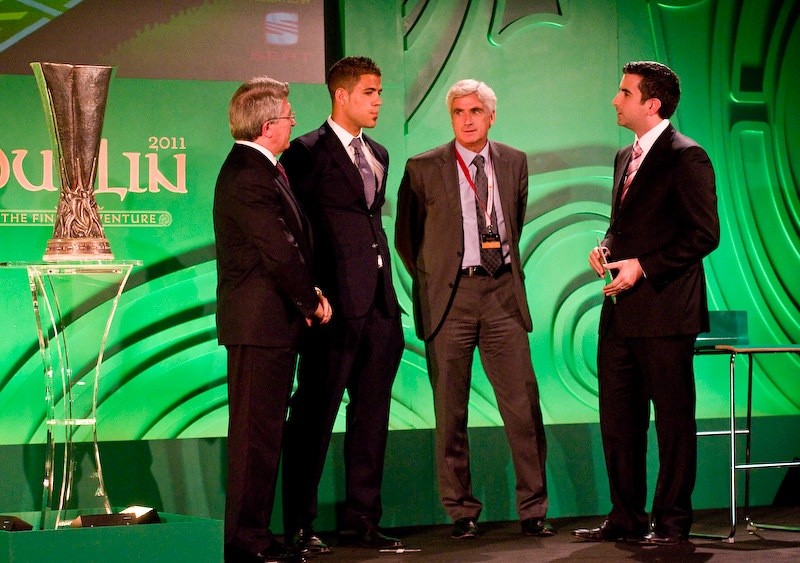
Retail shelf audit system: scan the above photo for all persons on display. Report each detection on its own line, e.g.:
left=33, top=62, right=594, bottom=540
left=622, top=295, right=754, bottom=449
left=211, top=72, right=335, bottom=562
left=394, top=77, right=558, bottom=540
left=279, top=56, right=412, bottom=558
left=567, top=61, right=721, bottom=546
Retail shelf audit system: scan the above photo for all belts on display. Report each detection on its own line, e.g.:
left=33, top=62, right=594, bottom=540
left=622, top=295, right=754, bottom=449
left=453, top=263, right=513, bottom=282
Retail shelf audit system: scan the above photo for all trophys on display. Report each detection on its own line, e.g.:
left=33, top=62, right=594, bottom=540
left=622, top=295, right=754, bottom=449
left=29, top=59, right=118, bottom=260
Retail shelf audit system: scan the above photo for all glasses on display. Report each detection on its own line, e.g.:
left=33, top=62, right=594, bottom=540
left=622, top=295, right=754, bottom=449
left=268, top=109, right=295, bottom=122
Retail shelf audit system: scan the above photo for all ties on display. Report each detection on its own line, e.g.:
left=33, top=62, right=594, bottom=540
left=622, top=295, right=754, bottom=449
left=470, top=153, right=503, bottom=277
left=350, top=136, right=377, bottom=213
left=275, top=161, right=292, bottom=198
left=617, top=140, right=643, bottom=216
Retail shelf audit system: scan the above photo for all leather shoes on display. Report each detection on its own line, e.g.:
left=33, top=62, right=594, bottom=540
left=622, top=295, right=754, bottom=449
left=522, top=514, right=557, bottom=541
left=450, top=515, right=483, bottom=541
left=292, top=528, right=334, bottom=556
left=257, top=540, right=305, bottom=563
left=337, top=524, right=406, bottom=550
left=572, top=509, right=649, bottom=547
left=618, top=521, right=694, bottom=552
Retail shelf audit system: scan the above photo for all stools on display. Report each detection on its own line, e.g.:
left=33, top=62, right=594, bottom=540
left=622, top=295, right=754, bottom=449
left=688, top=313, right=800, bottom=541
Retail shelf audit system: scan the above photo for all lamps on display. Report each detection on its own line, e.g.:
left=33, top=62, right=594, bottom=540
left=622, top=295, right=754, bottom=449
left=69, top=512, right=138, bottom=528
left=0, top=515, right=34, bottom=532
left=119, top=505, right=162, bottom=524
